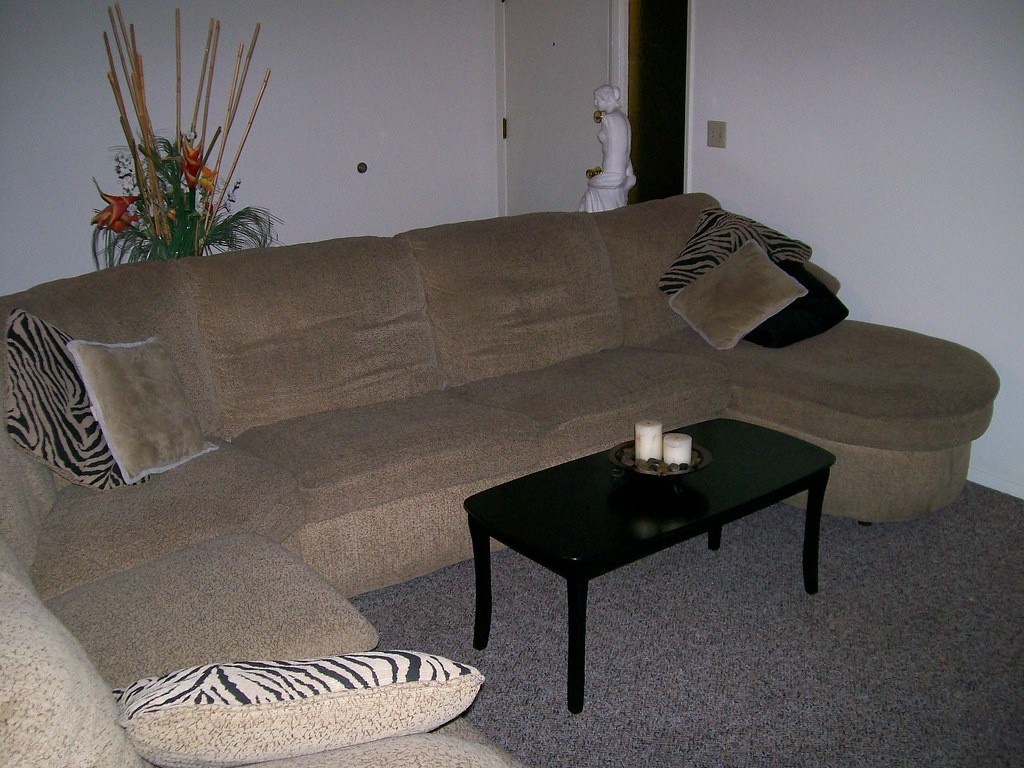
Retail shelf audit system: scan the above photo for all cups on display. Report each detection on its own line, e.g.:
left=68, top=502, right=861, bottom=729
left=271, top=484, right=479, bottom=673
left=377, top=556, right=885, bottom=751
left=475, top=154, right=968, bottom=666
left=664, top=432, right=692, bottom=466
left=634, top=422, right=662, bottom=461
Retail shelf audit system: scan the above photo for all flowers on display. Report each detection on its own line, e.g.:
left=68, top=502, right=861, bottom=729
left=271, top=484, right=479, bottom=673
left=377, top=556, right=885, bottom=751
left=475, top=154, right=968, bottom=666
left=89, top=122, right=285, bottom=273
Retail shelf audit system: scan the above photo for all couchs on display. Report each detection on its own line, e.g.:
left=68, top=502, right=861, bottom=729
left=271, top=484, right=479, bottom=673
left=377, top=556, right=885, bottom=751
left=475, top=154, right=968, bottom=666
left=0, top=192, right=1001, bottom=768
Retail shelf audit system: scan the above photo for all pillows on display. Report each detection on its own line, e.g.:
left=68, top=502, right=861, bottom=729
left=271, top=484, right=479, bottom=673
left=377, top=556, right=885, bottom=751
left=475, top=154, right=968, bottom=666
left=657, top=209, right=849, bottom=350
left=0, top=309, right=220, bottom=493
left=113, top=648, right=485, bottom=768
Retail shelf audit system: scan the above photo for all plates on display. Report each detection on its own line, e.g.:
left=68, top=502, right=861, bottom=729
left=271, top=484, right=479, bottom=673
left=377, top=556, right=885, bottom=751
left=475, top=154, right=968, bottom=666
left=610, top=441, right=712, bottom=476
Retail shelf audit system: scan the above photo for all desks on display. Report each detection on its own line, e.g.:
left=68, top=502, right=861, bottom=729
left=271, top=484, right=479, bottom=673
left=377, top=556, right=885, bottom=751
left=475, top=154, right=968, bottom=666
left=463, top=417, right=838, bottom=716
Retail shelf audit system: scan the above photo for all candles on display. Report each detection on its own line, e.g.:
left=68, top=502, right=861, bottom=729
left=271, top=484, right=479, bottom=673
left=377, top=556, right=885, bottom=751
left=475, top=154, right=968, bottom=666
left=635, top=420, right=663, bottom=460
left=663, top=434, right=692, bottom=465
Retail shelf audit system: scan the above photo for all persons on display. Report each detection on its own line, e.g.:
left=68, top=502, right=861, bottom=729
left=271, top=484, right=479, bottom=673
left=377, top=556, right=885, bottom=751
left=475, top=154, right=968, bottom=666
left=578, top=84, right=636, bottom=212
